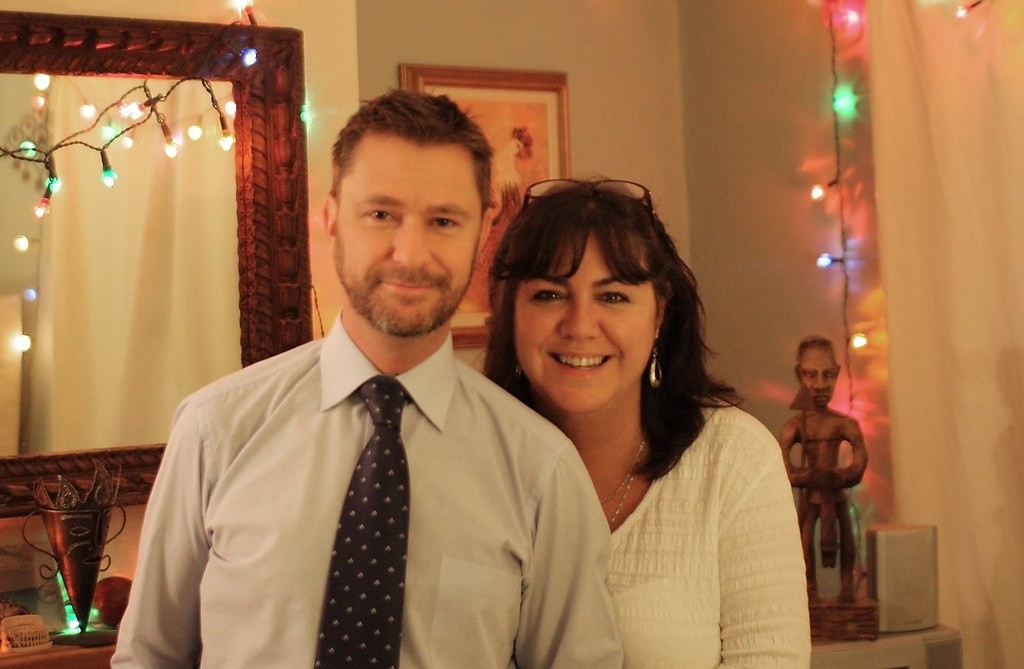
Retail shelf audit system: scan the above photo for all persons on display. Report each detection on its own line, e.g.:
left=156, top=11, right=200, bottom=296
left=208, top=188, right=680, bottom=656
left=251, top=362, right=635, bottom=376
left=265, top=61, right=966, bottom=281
left=111, top=86, right=625, bottom=669
left=779, top=336, right=868, bottom=607
left=480, top=176, right=812, bottom=669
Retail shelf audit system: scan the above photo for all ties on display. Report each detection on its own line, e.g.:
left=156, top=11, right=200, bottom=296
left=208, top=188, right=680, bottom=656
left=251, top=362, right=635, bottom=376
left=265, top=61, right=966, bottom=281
left=315, top=374, right=414, bottom=669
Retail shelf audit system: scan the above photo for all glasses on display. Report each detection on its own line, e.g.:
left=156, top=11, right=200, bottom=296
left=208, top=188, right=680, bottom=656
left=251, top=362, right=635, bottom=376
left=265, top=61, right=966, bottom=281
left=523, top=178, right=656, bottom=217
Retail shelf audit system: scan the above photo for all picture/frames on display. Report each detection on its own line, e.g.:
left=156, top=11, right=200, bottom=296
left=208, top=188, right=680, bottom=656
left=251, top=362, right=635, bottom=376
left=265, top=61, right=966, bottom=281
left=397, top=62, right=573, bottom=350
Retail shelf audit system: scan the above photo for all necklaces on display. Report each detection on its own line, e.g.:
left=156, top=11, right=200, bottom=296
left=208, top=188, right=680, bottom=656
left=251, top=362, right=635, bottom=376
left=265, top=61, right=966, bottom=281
left=600, top=438, right=643, bottom=527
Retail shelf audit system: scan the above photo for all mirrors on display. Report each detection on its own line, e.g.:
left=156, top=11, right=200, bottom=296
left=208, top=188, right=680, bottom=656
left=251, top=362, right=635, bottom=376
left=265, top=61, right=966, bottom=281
left=0, top=11, right=314, bottom=518
